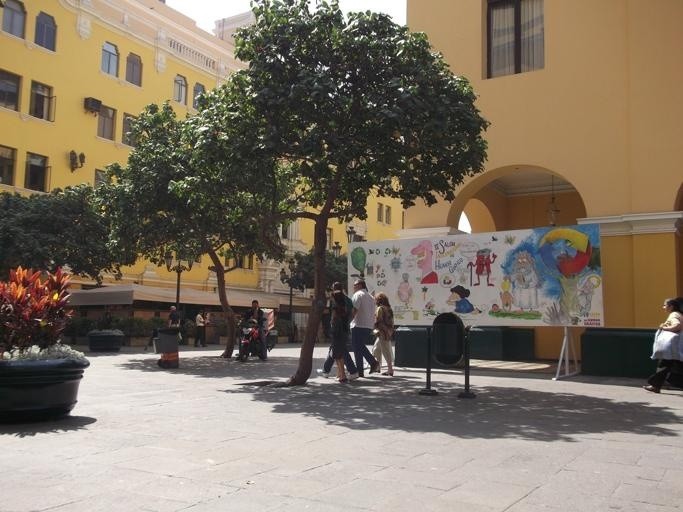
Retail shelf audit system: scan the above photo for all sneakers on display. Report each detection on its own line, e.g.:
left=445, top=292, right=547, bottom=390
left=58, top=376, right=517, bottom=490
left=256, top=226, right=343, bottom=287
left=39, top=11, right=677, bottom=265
left=369, top=362, right=393, bottom=375
left=317, top=369, right=329, bottom=377
left=339, top=373, right=359, bottom=381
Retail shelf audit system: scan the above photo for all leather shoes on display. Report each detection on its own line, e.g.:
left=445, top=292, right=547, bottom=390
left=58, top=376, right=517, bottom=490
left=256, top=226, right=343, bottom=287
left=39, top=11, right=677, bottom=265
left=643, top=385, right=660, bottom=393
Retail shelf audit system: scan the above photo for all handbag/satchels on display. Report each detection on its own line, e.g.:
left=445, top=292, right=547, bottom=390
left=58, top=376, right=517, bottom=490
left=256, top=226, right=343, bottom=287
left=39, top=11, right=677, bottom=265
left=651, top=317, right=683, bottom=361
left=367, top=336, right=375, bottom=345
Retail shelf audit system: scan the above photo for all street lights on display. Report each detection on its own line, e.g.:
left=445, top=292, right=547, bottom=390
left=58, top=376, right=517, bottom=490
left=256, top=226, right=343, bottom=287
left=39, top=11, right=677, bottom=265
left=164, top=251, right=193, bottom=313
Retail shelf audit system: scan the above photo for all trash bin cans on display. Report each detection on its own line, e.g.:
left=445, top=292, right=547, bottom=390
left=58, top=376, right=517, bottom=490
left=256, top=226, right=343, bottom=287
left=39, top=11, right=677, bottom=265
left=154, top=325, right=180, bottom=368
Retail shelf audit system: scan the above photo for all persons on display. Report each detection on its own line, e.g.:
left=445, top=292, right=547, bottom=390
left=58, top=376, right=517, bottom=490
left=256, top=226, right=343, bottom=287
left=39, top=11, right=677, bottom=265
left=194, top=309, right=210, bottom=347
left=371, top=292, right=393, bottom=376
left=350, top=279, right=378, bottom=377
left=642, top=298, right=683, bottom=393
left=168, top=306, right=183, bottom=344
left=237, top=301, right=268, bottom=361
left=316, top=289, right=357, bottom=378
left=330, top=281, right=349, bottom=385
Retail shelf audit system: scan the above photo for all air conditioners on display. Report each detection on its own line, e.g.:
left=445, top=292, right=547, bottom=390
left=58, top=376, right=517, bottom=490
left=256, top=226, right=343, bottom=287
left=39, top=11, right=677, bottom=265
left=85, top=97, right=101, bottom=117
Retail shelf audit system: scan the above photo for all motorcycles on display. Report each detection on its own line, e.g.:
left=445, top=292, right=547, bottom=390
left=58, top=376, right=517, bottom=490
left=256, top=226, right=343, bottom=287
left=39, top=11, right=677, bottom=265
left=237, top=319, right=270, bottom=362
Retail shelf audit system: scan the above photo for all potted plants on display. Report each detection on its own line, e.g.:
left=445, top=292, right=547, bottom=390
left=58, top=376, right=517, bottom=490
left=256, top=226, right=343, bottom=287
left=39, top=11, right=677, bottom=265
left=213, top=323, right=233, bottom=344
left=0, top=264, right=90, bottom=423
left=275, top=320, right=290, bottom=344
left=61, top=313, right=201, bottom=355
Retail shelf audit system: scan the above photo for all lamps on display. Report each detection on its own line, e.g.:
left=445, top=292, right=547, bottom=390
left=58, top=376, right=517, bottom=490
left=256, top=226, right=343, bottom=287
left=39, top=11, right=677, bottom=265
left=544, top=174, right=559, bottom=226
left=70, top=150, right=85, bottom=172
